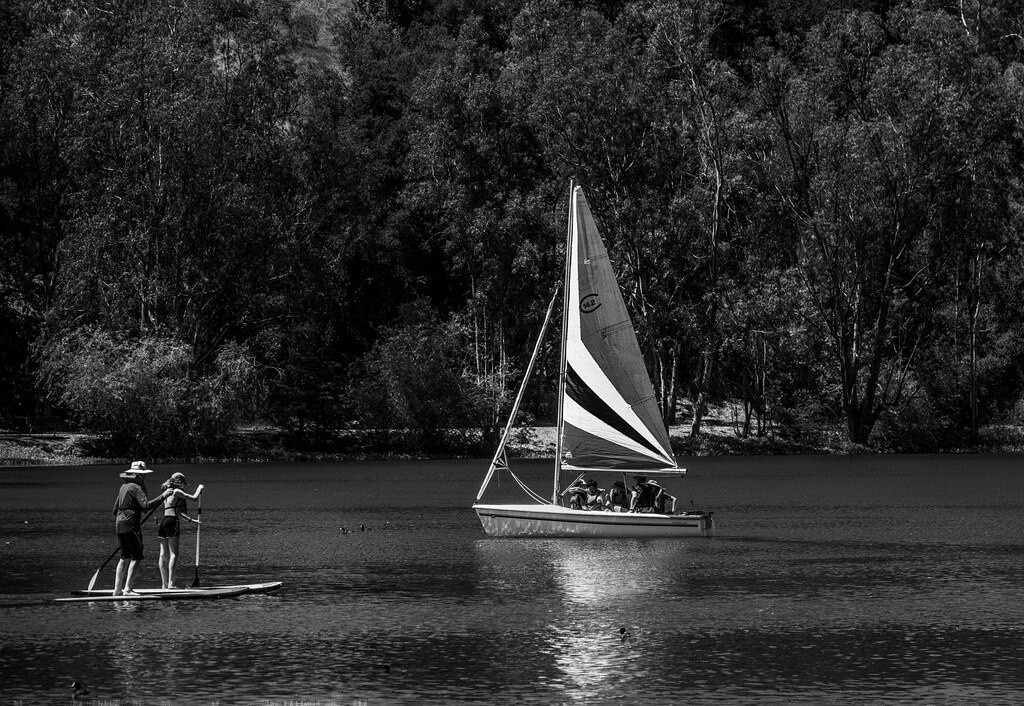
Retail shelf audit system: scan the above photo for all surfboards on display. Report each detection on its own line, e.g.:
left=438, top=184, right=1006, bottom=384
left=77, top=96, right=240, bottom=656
left=48, top=579, right=284, bottom=604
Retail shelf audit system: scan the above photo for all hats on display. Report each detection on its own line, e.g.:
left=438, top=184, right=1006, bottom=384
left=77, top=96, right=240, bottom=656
left=646, top=479, right=661, bottom=487
left=610, top=480, right=625, bottom=491
left=125, top=460, right=154, bottom=473
left=170, top=472, right=192, bottom=488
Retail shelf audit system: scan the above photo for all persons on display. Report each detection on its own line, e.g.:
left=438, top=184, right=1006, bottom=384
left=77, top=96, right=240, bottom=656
left=158, top=472, right=204, bottom=589
left=113, top=461, right=174, bottom=596
left=569, top=476, right=677, bottom=515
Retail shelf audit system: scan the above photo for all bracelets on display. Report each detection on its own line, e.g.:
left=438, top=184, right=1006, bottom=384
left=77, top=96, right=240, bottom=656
left=188, top=517, right=194, bottom=522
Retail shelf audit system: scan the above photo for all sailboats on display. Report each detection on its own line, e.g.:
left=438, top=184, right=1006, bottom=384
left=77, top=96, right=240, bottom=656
left=467, top=178, right=718, bottom=540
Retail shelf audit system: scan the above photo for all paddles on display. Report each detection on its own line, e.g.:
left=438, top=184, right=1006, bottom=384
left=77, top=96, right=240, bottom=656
left=190, top=489, right=203, bottom=589
left=87, top=491, right=171, bottom=592
